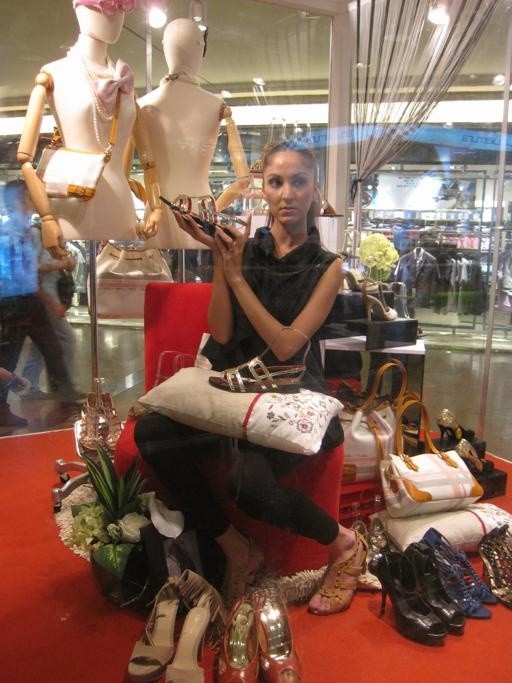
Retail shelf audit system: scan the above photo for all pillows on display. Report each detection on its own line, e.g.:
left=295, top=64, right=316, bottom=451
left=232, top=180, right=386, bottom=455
left=137, top=365, right=346, bottom=457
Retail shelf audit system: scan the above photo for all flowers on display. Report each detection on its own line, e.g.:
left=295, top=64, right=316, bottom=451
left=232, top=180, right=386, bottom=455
left=53, top=443, right=187, bottom=579
left=358, top=232, right=400, bottom=312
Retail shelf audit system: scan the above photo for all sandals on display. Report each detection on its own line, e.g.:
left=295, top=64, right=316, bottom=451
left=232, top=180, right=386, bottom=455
left=208, top=327, right=311, bottom=393
left=80, top=392, right=105, bottom=451
left=96, top=394, right=124, bottom=454
left=159, top=193, right=261, bottom=245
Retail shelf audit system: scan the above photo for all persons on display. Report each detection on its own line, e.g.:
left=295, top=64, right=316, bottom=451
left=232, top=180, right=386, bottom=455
left=0, top=181, right=86, bottom=429
left=133, top=142, right=369, bottom=616
left=18, top=1, right=142, bottom=259
left=1, top=366, right=29, bottom=391
left=18, top=221, right=90, bottom=402
left=124, top=18, right=251, bottom=248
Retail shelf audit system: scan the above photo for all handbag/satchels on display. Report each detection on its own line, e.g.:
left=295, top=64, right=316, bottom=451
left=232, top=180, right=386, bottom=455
left=35, top=146, right=110, bottom=201
left=87, top=242, right=174, bottom=318
left=380, top=449, right=483, bottom=517
left=337, top=401, right=395, bottom=484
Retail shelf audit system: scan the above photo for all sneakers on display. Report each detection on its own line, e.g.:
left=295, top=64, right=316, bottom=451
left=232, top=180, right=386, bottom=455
left=21, top=389, right=52, bottom=399
left=60, top=392, right=91, bottom=408
left=1, top=404, right=26, bottom=426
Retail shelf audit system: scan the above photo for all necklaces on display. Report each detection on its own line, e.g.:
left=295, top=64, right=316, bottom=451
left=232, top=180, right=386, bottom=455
left=67, top=47, right=118, bottom=149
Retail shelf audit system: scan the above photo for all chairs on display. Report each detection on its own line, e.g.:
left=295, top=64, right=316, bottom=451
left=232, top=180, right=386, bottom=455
left=111, top=281, right=344, bottom=571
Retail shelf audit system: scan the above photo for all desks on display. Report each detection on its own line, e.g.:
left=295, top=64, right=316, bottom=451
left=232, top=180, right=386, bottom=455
left=320, top=334, right=425, bottom=401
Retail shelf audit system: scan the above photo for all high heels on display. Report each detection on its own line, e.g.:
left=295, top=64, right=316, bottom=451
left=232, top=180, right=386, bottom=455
left=128, top=538, right=299, bottom=681
left=309, top=520, right=512, bottom=640
left=362, top=278, right=397, bottom=322
left=340, top=252, right=378, bottom=292
left=455, top=439, right=494, bottom=475
left=437, top=408, right=475, bottom=442
